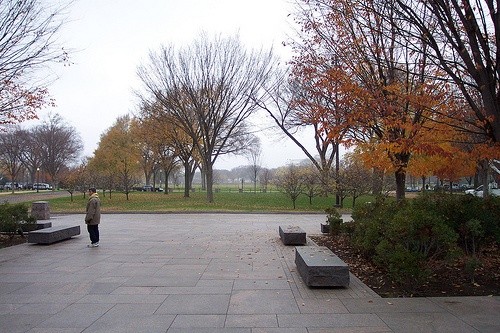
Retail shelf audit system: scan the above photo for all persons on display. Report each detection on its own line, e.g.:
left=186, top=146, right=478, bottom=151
left=85, top=187, right=101, bottom=247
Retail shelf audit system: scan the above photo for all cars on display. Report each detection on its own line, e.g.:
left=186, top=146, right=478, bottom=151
left=137, top=185, right=164, bottom=192
left=0, top=182, right=53, bottom=190
left=405, top=183, right=500, bottom=198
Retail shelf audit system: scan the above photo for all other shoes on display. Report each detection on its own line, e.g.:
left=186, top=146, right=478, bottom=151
left=87, top=242, right=99, bottom=248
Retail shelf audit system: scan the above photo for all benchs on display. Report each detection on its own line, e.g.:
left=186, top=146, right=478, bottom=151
left=321, top=223, right=329, bottom=232
left=295, top=246, right=349, bottom=286
left=279, top=225, right=306, bottom=244
left=28, top=225, right=80, bottom=243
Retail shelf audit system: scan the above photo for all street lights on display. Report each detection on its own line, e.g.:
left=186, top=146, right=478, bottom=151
left=36, top=168, right=40, bottom=193
left=159, top=169, right=162, bottom=188
